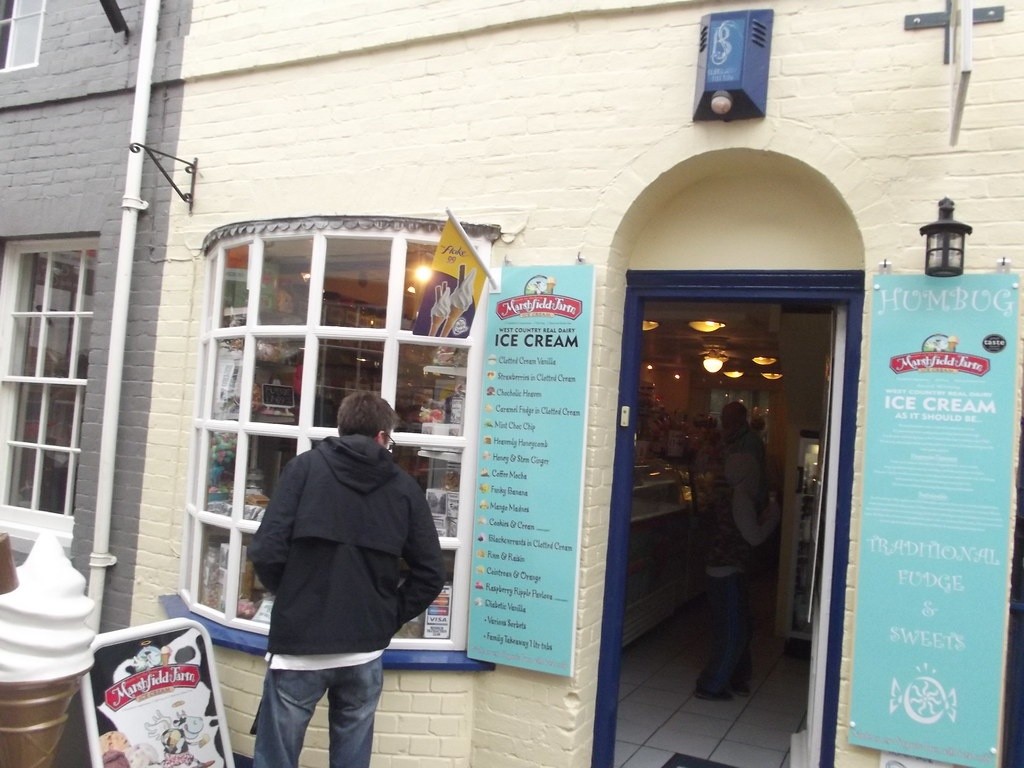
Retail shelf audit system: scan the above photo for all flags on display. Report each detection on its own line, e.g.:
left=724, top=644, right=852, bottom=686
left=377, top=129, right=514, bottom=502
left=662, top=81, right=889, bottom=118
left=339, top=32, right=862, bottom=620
left=413, top=218, right=486, bottom=339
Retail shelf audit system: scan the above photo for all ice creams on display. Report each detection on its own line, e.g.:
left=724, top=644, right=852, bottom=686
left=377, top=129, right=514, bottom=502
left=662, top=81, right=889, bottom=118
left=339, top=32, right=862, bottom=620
left=0, top=532, right=97, bottom=768
left=427, top=263, right=478, bottom=337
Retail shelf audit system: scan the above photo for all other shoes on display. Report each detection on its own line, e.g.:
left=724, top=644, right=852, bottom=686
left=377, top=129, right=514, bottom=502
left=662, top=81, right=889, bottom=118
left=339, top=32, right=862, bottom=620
left=693, top=667, right=751, bottom=702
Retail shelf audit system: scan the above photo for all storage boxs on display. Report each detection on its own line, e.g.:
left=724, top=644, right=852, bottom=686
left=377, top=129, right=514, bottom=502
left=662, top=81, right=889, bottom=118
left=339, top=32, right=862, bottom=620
left=200, top=488, right=270, bottom=620
left=412, top=482, right=458, bottom=638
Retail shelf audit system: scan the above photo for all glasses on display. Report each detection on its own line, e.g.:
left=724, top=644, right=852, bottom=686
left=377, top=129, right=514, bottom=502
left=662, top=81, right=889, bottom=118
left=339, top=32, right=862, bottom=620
left=384, top=431, right=396, bottom=453
left=719, top=413, right=735, bottom=420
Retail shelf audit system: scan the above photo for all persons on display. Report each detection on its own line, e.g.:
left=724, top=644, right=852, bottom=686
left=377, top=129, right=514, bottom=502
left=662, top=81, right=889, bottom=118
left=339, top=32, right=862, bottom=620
left=246, top=392, right=447, bottom=768
left=693, top=401, right=782, bottom=701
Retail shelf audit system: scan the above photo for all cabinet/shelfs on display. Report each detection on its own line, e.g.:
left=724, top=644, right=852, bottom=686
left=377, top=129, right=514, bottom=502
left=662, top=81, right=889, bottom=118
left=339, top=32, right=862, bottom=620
left=314, top=300, right=384, bottom=428
left=412, top=364, right=466, bottom=464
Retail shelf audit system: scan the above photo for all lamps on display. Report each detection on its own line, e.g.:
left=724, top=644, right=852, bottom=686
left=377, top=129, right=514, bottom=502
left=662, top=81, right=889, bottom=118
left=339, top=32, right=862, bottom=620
left=643, top=317, right=783, bottom=380
left=919, top=195, right=973, bottom=278
